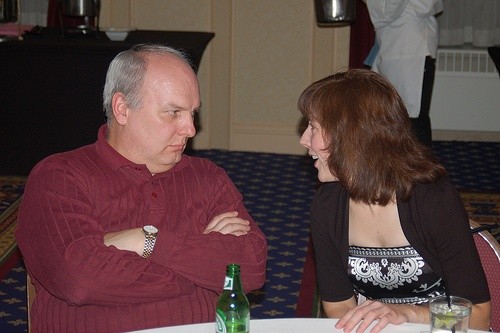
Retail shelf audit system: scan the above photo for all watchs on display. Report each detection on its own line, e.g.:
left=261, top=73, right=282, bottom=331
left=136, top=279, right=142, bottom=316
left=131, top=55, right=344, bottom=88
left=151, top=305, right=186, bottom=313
left=142, top=224, right=158, bottom=258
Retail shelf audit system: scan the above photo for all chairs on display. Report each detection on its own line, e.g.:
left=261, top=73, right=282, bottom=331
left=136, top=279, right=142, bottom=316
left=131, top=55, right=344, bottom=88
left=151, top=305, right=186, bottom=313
left=26, top=272, right=36, bottom=332
left=312, top=218, right=500, bottom=333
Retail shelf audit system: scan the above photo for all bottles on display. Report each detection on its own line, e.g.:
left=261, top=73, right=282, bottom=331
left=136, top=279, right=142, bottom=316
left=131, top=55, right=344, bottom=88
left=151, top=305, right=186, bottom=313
left=215, top=264, right=251, bottom=333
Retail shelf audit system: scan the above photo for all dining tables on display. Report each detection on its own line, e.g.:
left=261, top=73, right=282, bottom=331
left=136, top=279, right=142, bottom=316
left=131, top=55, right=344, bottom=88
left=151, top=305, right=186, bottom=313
left=126, top=318, right=490, bottom=332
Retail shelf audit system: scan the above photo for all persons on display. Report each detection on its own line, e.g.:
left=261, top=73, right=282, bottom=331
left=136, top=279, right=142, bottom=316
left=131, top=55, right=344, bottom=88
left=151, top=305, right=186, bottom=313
left=16, top=44, right=268, bottom=333
left=298, top=67, right=491, bottom=333
left=365, top=0, right=444, bottom=149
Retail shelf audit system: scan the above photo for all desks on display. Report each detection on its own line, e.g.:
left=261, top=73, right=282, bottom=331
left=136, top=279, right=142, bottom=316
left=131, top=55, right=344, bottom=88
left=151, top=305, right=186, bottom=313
left=0, top=25, right=215, bottom=176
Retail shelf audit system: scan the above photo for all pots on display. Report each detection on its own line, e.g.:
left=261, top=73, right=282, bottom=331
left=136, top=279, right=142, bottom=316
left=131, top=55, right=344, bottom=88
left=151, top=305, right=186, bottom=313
left=60, top=0, right=97, bottom=17
left=315, top=0, right=355, bottom=28
left=0, top=0, right=17, bottom=23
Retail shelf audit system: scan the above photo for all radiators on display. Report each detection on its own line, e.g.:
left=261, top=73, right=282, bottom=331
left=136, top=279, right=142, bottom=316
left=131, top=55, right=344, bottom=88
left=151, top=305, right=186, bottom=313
left=428, top=49, right=500, bottom=132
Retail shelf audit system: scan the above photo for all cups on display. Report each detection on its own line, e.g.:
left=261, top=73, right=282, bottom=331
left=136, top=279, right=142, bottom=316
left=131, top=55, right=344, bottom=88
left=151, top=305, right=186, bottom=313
left=428, top=295, right=473, bottom=333
